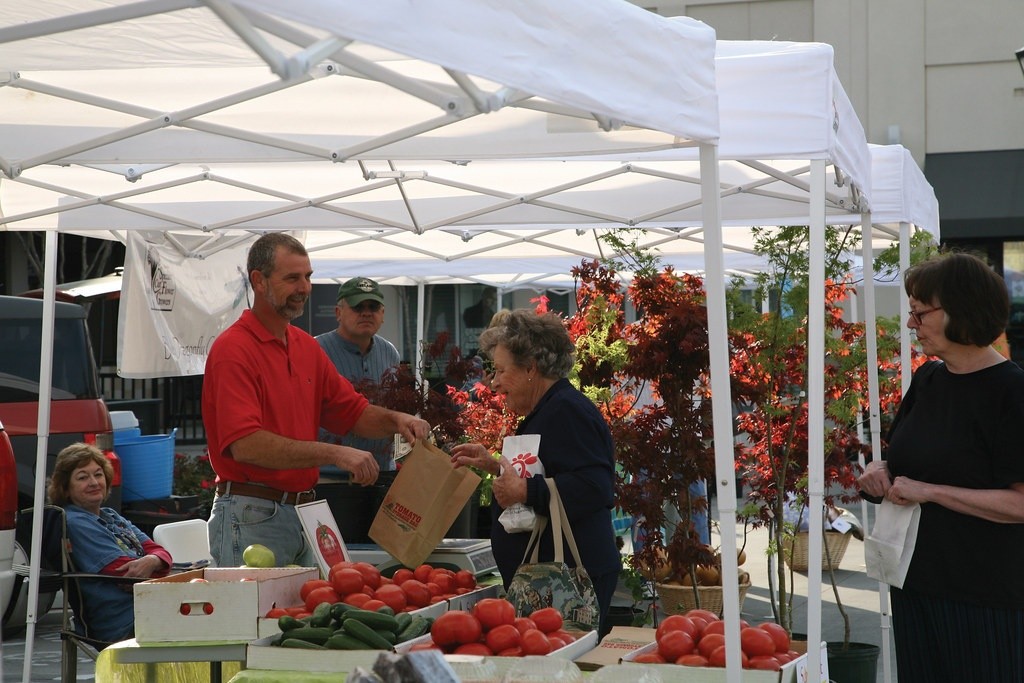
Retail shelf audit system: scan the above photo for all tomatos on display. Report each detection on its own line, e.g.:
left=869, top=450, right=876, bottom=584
left=264, top=561, right=482, bottom=620
left=180, top=604, right=190, bottom=615
left=410, top=599, right=577, bottom=657
left=204, top=603, right=213, bottom=614
left=635, top=610, right=801, bottom=671
left=190, top=578, right=208, bottom=583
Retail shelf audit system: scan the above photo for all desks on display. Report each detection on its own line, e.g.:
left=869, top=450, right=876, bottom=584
left=95, top=637, right=248, bottom=683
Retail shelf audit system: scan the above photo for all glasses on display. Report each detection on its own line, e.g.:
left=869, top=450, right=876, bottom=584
left=908, top=307, right=943, bottom=325
left=347, top=300, right=384, bottom=313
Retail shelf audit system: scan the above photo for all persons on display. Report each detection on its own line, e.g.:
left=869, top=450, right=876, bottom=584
left=448, top=308, right=622, bottom=639
left=46, top=440, right=173, bottom=654
left=201, top=234, right=431, bottom=566
left=852, top=254, right=1024, bottom=683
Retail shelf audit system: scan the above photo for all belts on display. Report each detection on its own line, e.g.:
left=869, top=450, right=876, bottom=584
left=216, top=481, right=314, bottom=504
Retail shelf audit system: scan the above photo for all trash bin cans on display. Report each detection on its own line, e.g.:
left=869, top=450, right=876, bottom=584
left=122, top=506, right=206, bottom=538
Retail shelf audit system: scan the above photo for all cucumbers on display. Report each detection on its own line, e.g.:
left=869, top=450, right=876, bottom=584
left=271, top=601, right=435, bottom=650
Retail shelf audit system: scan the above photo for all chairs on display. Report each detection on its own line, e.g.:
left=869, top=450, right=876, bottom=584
left=60, top=508, right=213, bottom=683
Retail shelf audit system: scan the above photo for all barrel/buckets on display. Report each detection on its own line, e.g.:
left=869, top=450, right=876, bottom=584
left=112, top=434, right=174, bottom=500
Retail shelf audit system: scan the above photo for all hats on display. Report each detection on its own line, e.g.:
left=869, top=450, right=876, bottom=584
left=336, top=276, right=385, bottom=308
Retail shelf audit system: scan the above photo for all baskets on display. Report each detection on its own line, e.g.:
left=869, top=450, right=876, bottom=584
left=654, top=567, right=752, bottom=617
left=783, top=532, right=853, bottom=570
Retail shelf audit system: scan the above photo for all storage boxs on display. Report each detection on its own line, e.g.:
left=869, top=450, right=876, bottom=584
left=133, top=567, right=830, bottom=683
left=108, top=409, right=142, bottom=439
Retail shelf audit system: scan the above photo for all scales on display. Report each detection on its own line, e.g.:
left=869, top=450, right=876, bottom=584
left=374, top=537, right=498, bottom=581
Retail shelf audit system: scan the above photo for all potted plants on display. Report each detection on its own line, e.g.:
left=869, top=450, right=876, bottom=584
left=446, top=226, right=940, bottom=683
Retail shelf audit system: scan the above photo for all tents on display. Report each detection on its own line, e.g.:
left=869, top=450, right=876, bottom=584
left=0, top=2, right=939, bottom=379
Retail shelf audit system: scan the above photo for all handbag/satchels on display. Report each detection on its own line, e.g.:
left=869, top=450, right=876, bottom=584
left=368, top=438, right=482, bottom=570
left=504, top=477, right=600, bottom=631
